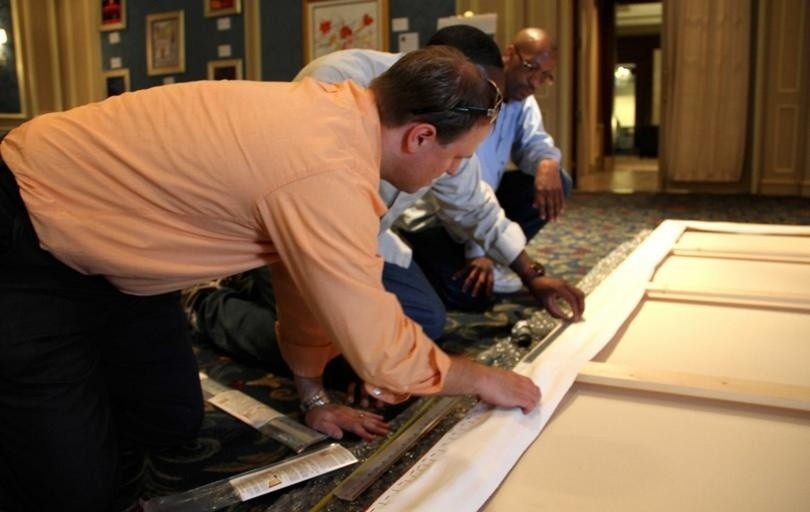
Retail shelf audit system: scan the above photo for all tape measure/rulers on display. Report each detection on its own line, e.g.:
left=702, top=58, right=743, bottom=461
left=334, top=394, right=466, bottom=502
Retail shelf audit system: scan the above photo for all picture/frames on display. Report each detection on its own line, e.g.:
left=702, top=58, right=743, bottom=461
left=0, top=1, right=28, bottom=120
left=302, top=0, right=390, bottom=70
left=99, top=0, right=243, bottom=100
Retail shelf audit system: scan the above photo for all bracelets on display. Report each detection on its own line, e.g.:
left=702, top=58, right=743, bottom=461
left=300, top=388, right=331, bottom=413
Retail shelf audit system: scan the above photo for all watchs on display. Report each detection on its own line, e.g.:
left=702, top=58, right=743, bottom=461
left=1, top=45, right=544, bottom=512
left=522, top=261, right=547, bottom=285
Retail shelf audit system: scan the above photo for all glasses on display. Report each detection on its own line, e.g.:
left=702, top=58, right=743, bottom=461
left=411, top=75, right=504, bottom=127
left=513, top=41, right=556, bottom=86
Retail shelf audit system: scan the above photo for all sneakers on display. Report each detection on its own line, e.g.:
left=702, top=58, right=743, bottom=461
left=492, top=263, right=523, bottom=294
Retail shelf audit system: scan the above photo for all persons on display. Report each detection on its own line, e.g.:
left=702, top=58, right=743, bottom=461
left=391, top=25, right=575, bottom=318
left=182, top=22, right=589, bottom=409
